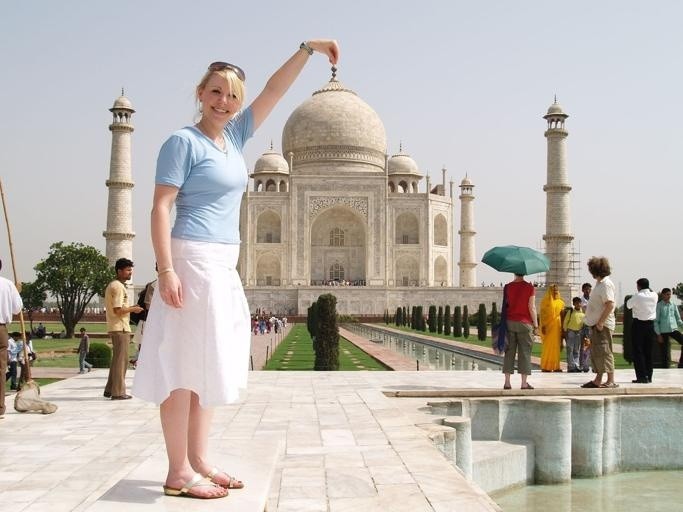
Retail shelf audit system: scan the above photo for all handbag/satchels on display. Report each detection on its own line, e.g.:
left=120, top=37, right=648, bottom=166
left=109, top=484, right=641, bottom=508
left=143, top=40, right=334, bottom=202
left=157, top=267, right=174, bottom=277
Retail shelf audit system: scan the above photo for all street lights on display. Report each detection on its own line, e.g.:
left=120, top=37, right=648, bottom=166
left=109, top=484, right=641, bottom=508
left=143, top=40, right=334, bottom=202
left=492, top=322, right=510, bottom=354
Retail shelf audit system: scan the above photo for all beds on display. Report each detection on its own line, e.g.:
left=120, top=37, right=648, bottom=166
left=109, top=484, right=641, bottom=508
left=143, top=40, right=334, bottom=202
left=481, top=244, right=552, bottom=275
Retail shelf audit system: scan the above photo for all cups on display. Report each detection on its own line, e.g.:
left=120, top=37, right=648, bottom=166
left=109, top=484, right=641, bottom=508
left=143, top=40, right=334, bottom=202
left=207, top=60, right=246, bottom=81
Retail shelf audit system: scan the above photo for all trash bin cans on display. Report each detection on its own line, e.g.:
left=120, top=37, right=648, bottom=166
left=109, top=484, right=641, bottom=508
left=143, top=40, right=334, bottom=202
left=299, top=40, right=313, bottom=56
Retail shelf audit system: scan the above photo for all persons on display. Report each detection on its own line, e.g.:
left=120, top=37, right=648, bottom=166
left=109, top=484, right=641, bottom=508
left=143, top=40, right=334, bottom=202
left=312, top=278, right=367, bottom=287
left=502, top=273, right=535, bottom=390
left=441, top=282, right=443, bottom=289
left=0, top=258, right=159, bottom=420
left=130, top=37, right=336, bottom=500
left=538, top=256, right=683, bottom=388
left=480, top=281, right=546, bottom=288
left=250, top=311, right=288, bottom=335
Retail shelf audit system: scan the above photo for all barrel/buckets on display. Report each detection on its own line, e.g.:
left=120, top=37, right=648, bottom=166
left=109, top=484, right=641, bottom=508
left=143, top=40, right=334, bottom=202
left=163, top=468, right=244, bottom=499
left=504, top=385, right=511, bottom=390
left=521, top=383, right=534, bottom=389
left=581, top=381, right=619, bottom=388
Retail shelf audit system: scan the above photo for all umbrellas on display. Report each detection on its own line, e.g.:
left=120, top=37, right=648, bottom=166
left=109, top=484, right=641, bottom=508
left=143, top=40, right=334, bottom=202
left=103, top=391, right=132, bottom=400
left=632, top=375, right=652, bottom=383
